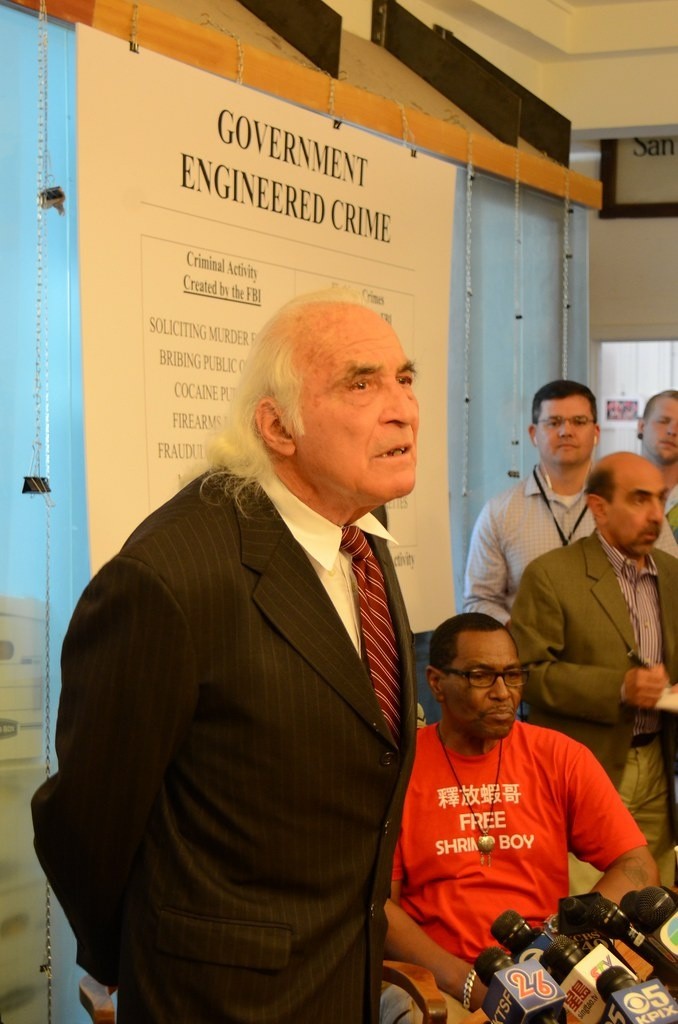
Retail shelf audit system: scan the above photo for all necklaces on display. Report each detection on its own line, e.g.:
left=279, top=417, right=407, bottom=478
left=436, top=719, right=503, bottom=866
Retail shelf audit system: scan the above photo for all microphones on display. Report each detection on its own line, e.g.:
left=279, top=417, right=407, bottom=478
left=469, top=885, right=678, bottom=1024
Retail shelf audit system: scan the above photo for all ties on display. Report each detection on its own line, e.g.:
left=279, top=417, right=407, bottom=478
left=341, top=526, right=402, bottom=741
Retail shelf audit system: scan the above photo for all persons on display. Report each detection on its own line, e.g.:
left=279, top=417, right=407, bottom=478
left=31, top=289, right=422, bottom=1024
left=637, top=390, right=678, bottom=543
left=378, top=614, right=661, bottom=1024
left=506, top=452, right=678, bottom=895
left=463, top=379, right=678, bottom=627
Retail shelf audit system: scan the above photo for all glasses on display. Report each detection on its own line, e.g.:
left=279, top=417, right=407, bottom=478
left=539, top=415, right=595, bottom=429
left=444, top=668, right=530, bottom=688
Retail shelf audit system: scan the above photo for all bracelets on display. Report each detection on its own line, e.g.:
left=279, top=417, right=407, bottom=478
left=463, top=968, right=477, bottom=1011
